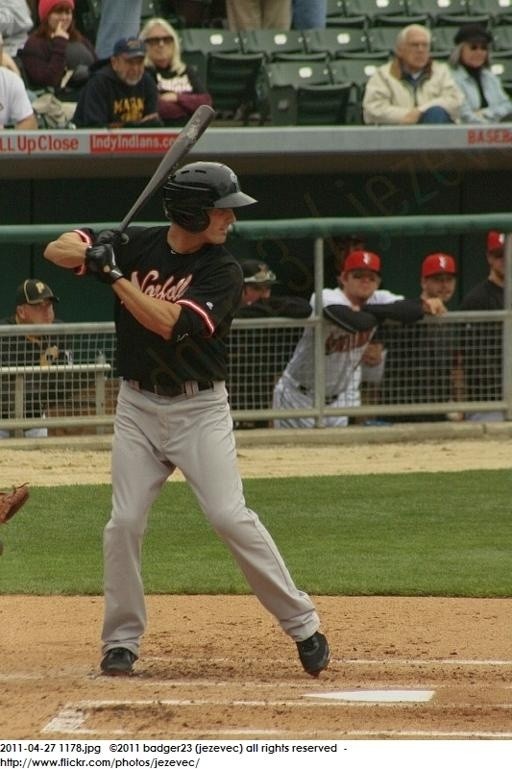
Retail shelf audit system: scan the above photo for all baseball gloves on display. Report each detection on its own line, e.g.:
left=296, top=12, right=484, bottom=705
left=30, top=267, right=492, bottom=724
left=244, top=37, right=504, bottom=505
left=1, top=484, right=28, bottom=520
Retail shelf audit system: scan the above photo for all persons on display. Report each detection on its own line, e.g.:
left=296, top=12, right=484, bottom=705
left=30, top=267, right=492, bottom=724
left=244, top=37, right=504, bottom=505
left=43, top=160, right=331, bottom=676
left=361, top=24, right=462, bottom=126
left=446, top=27, right=512, bottom=125
left=398, top=247, right=465, bottom=419
left=273, top=251, right=408, bottom=430
left=461, top=230, right=506, bottom=403
left=0, top=279, right=73, bottom=411
left=0, top=0, right=213, bottom=130
left=232, top=259, right=312, bottom=317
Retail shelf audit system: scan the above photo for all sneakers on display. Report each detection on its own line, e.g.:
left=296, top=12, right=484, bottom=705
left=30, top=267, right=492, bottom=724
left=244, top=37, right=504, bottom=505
left=100, top=648, right=138, bottom=674
left=295, top=626, right=331, bottom=676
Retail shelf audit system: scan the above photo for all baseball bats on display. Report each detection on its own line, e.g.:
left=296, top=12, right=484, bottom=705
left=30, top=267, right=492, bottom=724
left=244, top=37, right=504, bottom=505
left=106, top=104, right=215, bottom=249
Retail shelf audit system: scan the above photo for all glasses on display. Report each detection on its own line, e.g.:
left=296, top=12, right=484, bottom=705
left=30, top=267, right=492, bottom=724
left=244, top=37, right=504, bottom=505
left=144, top=36, right=174, bottom=46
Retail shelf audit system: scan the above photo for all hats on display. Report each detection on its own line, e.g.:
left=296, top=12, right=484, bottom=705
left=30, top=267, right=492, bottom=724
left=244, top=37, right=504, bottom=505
left=38, top=0, right=146, bottom=62
left=454, top=25, right=493, bottom=44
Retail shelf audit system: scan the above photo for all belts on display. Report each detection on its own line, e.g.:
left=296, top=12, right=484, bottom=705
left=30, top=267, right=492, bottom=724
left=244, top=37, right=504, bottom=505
left=122, top=375, right=213, bottom=397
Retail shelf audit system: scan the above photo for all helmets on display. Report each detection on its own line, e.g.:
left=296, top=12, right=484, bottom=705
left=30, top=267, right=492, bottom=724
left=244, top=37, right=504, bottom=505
left=163, top=161, right=260, bottom=234
